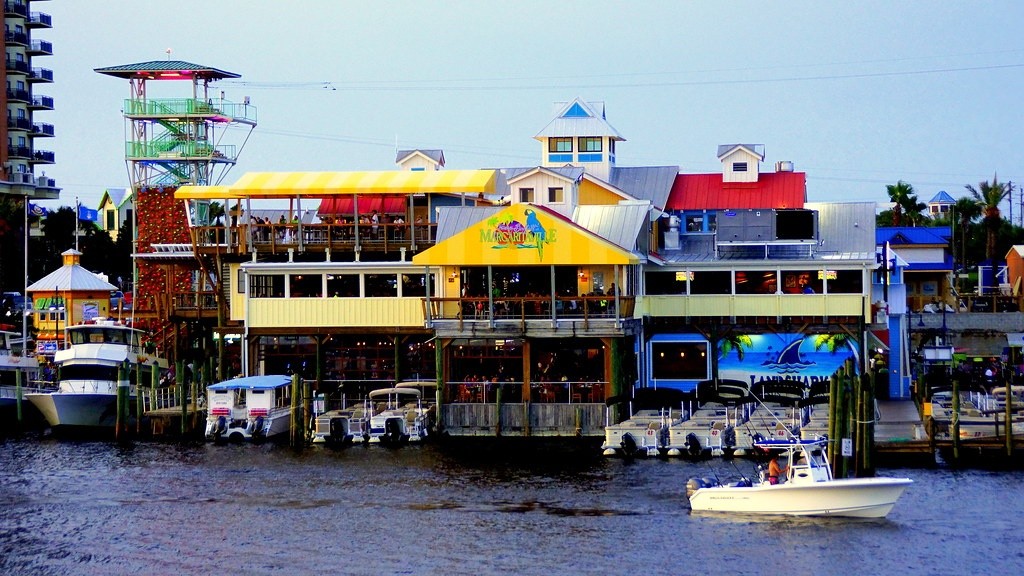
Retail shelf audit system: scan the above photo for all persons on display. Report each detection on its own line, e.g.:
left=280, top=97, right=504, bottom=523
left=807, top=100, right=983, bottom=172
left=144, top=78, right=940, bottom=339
left=461, top=374, right=604, bottom=403
left=768, top=453, right=790, bottom=485
left=159, top=355, right=242, bottom=398
left=956, top=360, right=998, bottom=388
left=235, top=210, right=425, bottom=241
left=797, top=451, right=807, bottom=465
left=43, top=360, right=63, bottom=382
left=460, top=278, right=622, bottom=315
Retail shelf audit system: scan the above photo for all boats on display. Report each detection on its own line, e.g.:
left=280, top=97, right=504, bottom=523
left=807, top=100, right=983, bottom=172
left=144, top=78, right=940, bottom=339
left=311, top=401, right=402, bottom=449
left=599, top=378, right=829, bottom=462
left=931, top=384, right=1024, bottom=446
left=0, top=329, right=58, bottom=434
left=22, top=316, right=175, bottom=437
left=685, top=387, right=915, bottom=523
left=365, top=380, right=435, bottom=450
left=202, top=373, right=304, bottom=445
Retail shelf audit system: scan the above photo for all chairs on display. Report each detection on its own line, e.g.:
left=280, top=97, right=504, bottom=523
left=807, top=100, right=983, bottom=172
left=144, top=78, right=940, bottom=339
left=243, top=226, right=425, bottom=242
left=476, top=384, right=488, bottom=403
left=571, top=387, right=581, bottom=403
left=351, top=408, right=364, bottom=422
left=407, top=409, right=417, bottom=425
left=474, top=299, right=614, bottom=319
left=539, top=385, right=556, bottom=403
left=588, top=385, right=600, bottom=402
left=458, top=384, right=470, bottom=402
